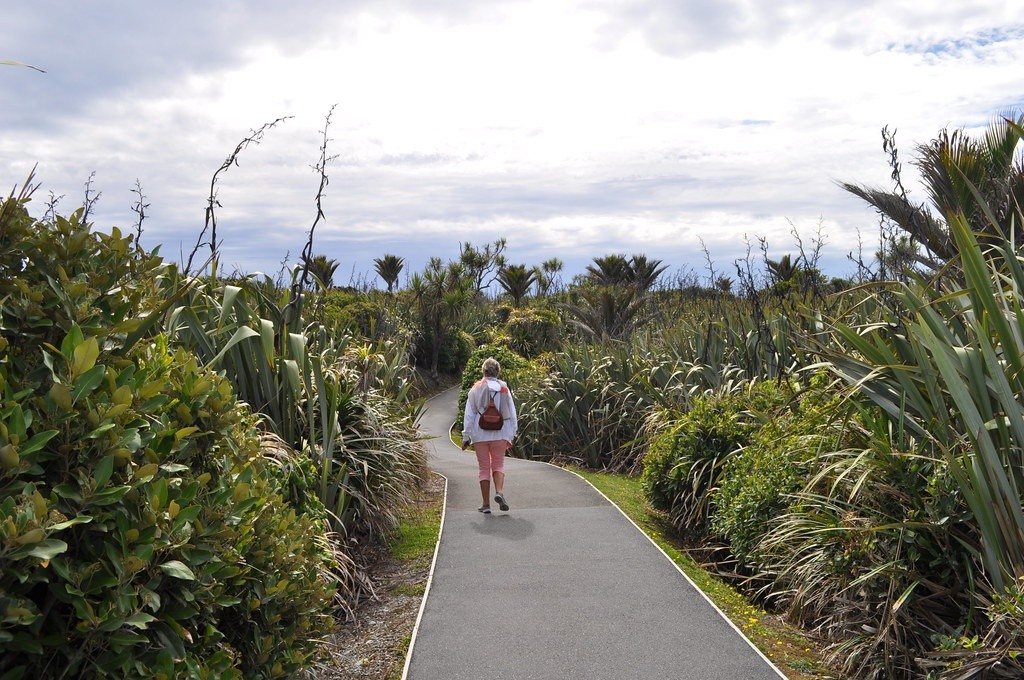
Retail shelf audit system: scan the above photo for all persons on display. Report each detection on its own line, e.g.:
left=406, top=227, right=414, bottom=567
left=461, top=358, right=518, bottom=513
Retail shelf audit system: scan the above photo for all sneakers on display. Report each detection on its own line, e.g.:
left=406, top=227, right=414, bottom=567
left=494, top=492, right=509, bottom=511
left=477, top=504, right=491, bottom=513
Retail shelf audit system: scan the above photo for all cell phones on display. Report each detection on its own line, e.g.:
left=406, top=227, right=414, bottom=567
left=463, top=441, right=469, bottom=450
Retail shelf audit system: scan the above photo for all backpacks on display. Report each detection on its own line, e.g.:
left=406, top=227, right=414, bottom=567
left=476, top=390, right=510, bottom=431
